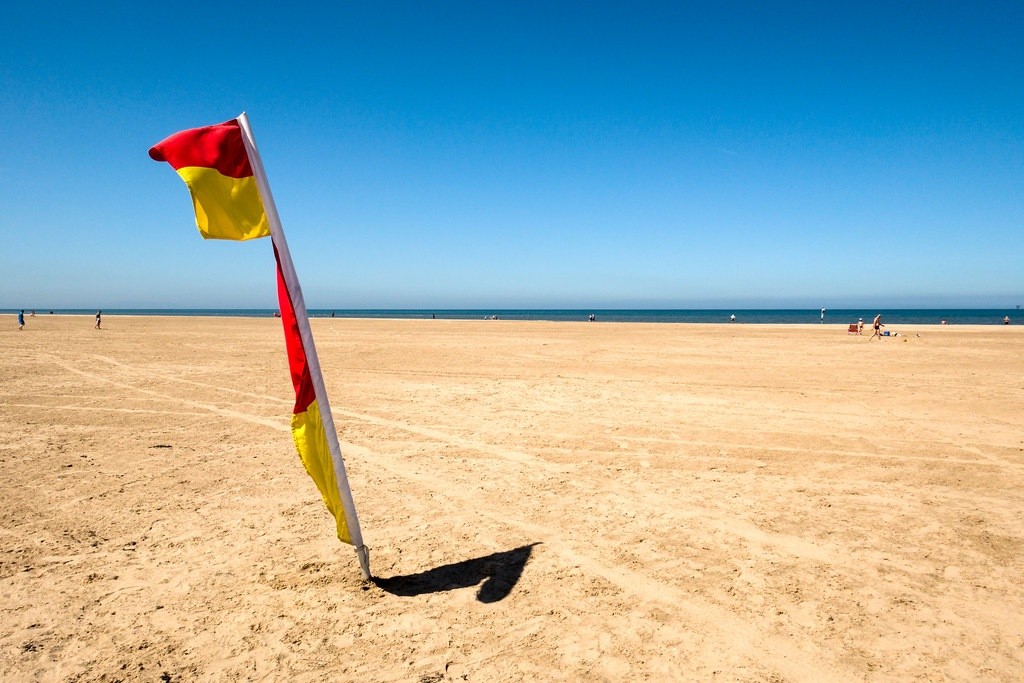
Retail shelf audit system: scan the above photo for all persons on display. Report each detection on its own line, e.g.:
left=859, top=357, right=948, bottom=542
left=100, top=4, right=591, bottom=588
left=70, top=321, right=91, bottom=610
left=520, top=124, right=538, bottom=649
left=18, top=309, right=25, bottom=330
left=588, top=313, right=595, bottom=322
left=858, top=318, right=864, bottom=336
left=485, top=315, right=497, bottom=320
left=1003, top=315, right=1010, bottom=325
left=869, top=314, right=884, bottom=341
left=95, top=310, right=101, bottom=330
left=30, top=309, right=36, bottom=317
left=273, top=312, right=281, bottom=317
left=941, top=319, right=946, bottom=325
left=432, top=313, right=435, bottom=319
left=731, top=314, right=736, bottom=323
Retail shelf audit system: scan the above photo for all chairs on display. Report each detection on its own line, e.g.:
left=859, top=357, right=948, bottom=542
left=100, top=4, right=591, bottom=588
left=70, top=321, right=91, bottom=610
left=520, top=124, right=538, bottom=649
left=848, top=324, right=858, bottom=335
